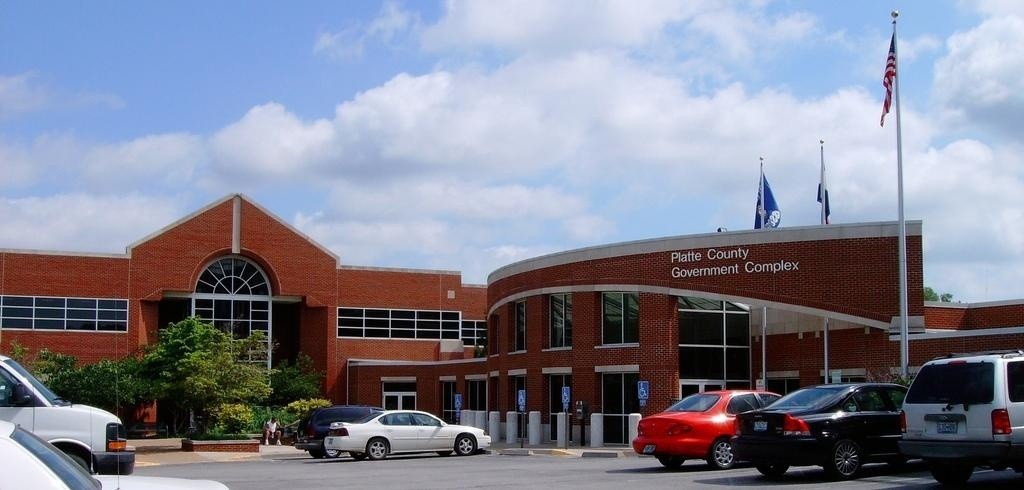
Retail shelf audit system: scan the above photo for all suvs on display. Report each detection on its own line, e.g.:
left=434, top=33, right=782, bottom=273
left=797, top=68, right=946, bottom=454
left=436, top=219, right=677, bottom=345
left=296, top=405, right=387, bottom=458
left=896, top=349, right=1024, bottom=485
left=0, top=356, right=136, bottom=477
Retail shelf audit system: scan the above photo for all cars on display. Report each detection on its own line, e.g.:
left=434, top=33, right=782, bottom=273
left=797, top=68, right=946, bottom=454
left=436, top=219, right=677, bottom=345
left=0, top=421, right=227, bottom=489
left=326, top=409, right=492, bottom=460
left=633, top=390, right=784, bottom=470
left=729, top=383, right=911, bottom=479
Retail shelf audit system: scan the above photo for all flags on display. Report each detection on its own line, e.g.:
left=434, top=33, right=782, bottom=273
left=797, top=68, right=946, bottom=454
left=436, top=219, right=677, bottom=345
left=754, top=174, right=780, bottom=229
left=880, top=31, right=896, bottom=127
left=817, top=158, right=830, bottom=224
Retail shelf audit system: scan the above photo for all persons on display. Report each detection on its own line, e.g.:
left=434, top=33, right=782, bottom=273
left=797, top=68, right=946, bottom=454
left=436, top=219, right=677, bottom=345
left=262, top=417, right=282, bottom=447
